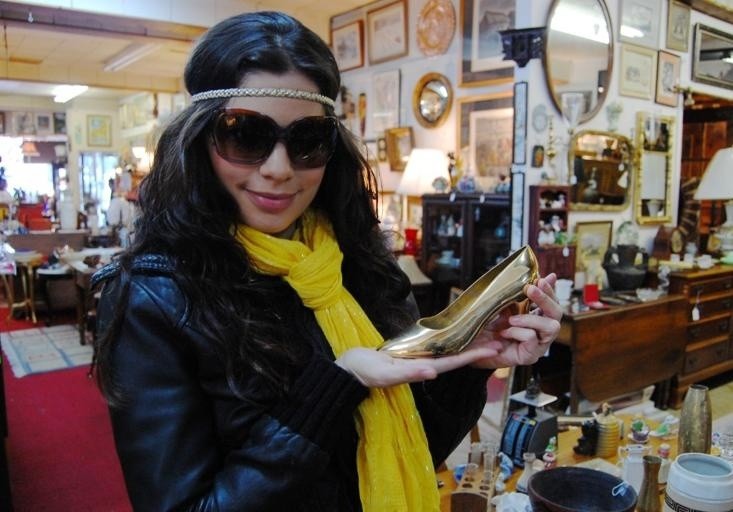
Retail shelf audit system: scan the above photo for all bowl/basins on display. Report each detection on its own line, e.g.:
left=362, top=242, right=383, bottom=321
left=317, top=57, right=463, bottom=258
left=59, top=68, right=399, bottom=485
left=635, top=288, right=662, bottom=303
left=526, top=464, right=637, bottom=511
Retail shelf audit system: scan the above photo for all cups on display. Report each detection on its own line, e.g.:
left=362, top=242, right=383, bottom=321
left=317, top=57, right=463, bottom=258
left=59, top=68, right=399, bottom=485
left=583, top=284, right=599, bottom=305
left=645, top=202, right=659, bottom=218
left=670, top=253, right=714, bottom=270
left=574, top=272, right=604, bottom=292
left=441, top=250, right=453, bottom=261
left=554, top=278, right=575, bottom=304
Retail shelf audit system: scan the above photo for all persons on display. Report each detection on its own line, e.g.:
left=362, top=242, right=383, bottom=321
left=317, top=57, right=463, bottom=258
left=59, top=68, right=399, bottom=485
left=86, top=11, right=564, bottom=512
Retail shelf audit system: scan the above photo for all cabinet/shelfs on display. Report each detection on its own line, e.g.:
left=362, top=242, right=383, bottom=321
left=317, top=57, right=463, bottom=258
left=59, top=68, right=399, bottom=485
left=649, top=272, right=732, bottom=409
left=530, top=185, right=575, bottom=279
left=682, top=122, right=728, bottom=237
left=423, top=194, right=510, bottom=311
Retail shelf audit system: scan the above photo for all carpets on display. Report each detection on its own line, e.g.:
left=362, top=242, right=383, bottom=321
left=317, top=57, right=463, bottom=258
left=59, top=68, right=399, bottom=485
left=0, top=325, right=96, bottom=379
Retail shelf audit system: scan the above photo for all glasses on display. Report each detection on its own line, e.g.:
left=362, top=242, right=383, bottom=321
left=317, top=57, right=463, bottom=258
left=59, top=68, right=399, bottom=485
left=206, top=106, right=341, bottom=171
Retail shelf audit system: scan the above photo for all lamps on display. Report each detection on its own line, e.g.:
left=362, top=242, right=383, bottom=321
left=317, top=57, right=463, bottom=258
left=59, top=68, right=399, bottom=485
left=103, top=42, right=160, bottom=71
left=642, top=151, right=668, bottom=216
left=22, top=142, right=40, bottom=157
left=693, top=147, right=733, bottom=256
left=398, top=256, right=431, bottom=284
left=398, top=149, right=451, bottom=197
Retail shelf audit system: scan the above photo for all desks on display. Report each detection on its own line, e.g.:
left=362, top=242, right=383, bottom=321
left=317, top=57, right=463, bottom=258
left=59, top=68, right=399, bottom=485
left=584, top=157, right=623, bottom=197
left=0, top=231, right=119, bottom=345
left=553, top=294, right=689, bottom=415
left=439, top=401, right=718, bottom=512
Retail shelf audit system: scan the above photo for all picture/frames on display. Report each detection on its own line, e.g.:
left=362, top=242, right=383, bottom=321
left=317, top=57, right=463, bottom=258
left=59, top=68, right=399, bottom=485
left=618, top=1, right=732, bottom=106
left=458, top=0, right=514, bottom=87
left=407, top=195, right=423, bottom=226
left=376, top=191, right=403, bottom=228
left=0, top=110, right=65, bottom=135
left=575, top=221, right=613, bottom=267
left=456, top=89, right=513, bottom=188
left=385, top=128, right=413, bottom=171
left=86, top=91, right=187, bottom=148
left=330, top=19, right=364, bottom=72
left=367, top=0, right=409, bottom=65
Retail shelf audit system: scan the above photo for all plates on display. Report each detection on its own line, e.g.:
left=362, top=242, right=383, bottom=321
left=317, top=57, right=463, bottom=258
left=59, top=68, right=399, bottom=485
left=416, top=0, right=455, bottom=57
left=434, top=257, right=452, bottom=265
left=456, top=176, right=477, bottom=192
left=430, top=176, right=450, bottom=191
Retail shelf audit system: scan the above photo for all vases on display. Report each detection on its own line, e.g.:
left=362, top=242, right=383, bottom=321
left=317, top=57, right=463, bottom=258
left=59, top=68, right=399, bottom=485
left=405, top=229, right=418, bottom=255
left=635, top=454, right=662, bottom=512
left=61, top=190, right=77, bottom=230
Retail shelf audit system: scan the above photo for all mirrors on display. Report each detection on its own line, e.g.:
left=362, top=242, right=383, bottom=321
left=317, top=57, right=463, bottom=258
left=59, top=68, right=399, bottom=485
left=568, top=129, right=634, bottom=212
left=542, top=0, right=614, bottom=125
left=635, top=111, right=676, bottom=224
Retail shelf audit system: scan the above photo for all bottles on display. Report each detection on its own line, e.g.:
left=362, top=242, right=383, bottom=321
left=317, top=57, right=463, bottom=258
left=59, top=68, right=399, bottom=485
left=663, top=452, right=733, bottom=512
left=653, top=443, right=672, bottom=485
left=465, top=440, right=497, bottom=480
left=538, top=194, right=567, bottom=246
left=594, top=404, right=620, bottom=459
left=622, top=444, right=644, bottom=492
left=517, top=452, right=557, bottom=492
left=638, top=455, right=663, bottom=512
left=438, top=214, right=463, bottom=237
left=405, top=228, right=419, bottom=257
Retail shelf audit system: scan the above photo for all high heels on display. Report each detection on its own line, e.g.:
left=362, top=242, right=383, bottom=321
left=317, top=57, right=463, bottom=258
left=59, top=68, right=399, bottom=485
left=373, top=242, right=541, bottom=361
